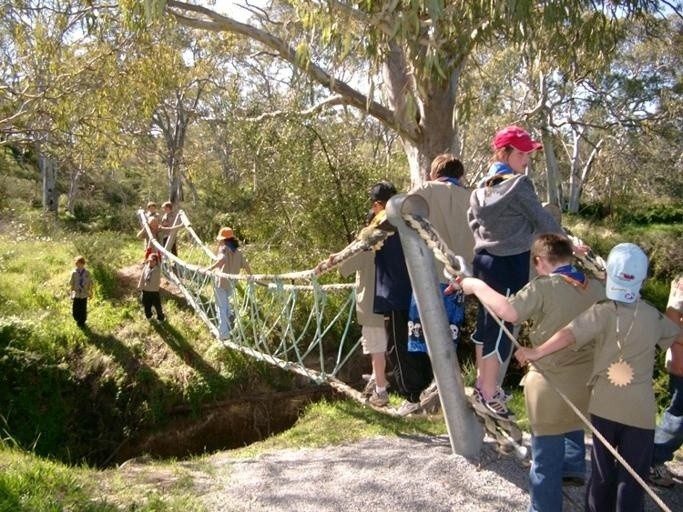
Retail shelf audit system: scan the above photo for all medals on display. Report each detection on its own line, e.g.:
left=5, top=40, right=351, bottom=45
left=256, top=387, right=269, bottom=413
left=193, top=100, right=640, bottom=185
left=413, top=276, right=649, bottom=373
left=606, top=360, right=634, bottom=387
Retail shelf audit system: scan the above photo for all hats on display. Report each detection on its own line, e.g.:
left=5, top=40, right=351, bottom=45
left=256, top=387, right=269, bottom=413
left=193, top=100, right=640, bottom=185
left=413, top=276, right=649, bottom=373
left=370, top=179, right=397, bottom=202
left=216, top=227, right=235, bottom=241
left=494, top=127, right=544, bottom=154
left=606, top=240, right=649, bottom=304
left=146, top=253, right=156, bottom=262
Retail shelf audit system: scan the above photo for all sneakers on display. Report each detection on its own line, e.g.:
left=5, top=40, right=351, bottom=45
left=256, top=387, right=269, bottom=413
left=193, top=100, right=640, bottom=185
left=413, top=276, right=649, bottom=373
left=472, top=380, right=514, bottom=403
left=561, top=469, right=586, bottom=488
left=157, top=316, right=166, bottom=324
left=147, top=315, right=153, bottom=321
left=472, top=388, right=511, bottom=422
left=368, top=390, right=390, bottom=407
left=648, top=461, right=675, bottom=488
left=361, top=374, right=391, bottom=398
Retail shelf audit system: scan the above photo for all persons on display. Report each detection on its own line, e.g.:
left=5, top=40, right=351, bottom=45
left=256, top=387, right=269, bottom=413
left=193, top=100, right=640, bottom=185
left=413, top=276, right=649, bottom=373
left=137, top=253, right=165, bottom=322
left=314, top=128, right=591, bottom=422
left=203, top=226, right=254, bottom=341
left=458, top=234, right=683, bottom=511
left=157, top=202, right=177, bottom=255
left=69, top=255, right=95, bottom=327
left=137, top=202, right=161, bottom=264
